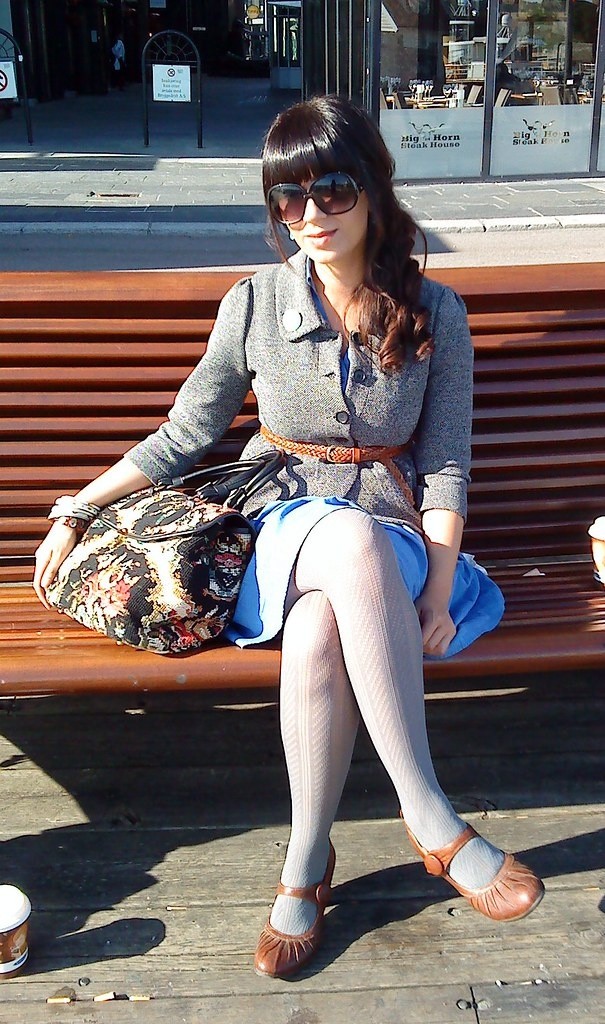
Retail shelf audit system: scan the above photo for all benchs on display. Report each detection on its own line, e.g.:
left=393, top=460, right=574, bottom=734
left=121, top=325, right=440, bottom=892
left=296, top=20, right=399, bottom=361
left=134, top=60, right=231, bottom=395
left=0, top=263, right=604, bottom=702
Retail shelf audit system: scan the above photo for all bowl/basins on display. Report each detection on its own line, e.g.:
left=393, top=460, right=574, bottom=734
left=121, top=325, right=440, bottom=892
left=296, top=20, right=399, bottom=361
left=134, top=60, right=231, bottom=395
left=587, top=515, right=604, bottom=582
left=0, top=884, right=32, bottom=973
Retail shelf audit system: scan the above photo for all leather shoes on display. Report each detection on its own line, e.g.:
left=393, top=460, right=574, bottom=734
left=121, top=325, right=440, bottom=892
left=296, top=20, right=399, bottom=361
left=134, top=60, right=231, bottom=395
left=253, top=836, right=337, bottom=979
left=398, top=799, right=544, bottom=922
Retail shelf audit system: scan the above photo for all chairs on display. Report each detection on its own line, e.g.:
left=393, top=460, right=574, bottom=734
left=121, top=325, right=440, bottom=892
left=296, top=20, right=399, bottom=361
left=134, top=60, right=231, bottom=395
left=379, top=83, right=579, bottom=110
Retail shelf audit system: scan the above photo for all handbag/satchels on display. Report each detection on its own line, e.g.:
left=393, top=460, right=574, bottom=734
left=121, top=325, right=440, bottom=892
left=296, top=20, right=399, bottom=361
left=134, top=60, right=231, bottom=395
left=44, top=447, right=288, bottom=655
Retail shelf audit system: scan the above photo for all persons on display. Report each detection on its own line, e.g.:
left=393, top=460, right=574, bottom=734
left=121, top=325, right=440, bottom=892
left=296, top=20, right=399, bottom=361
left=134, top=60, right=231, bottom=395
left=112, top=39, right=127, bottom=92
left=31, top=92, right=546, bottom=978
left=495, top=62, right=522, bottom=97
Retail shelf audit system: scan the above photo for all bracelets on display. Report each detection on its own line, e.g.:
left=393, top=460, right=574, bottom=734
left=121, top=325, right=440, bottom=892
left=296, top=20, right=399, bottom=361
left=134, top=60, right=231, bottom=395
left=47, top=494, right=100, bottom=530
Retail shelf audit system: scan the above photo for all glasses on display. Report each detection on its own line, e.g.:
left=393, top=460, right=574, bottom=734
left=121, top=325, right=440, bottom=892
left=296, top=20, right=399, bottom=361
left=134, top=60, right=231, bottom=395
left=266, top=171, right=365, bottom=223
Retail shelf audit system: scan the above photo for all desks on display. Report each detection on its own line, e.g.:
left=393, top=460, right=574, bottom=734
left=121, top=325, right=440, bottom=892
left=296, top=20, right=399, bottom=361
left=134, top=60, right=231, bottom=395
left=522, top=93, right=545, bottom=105
